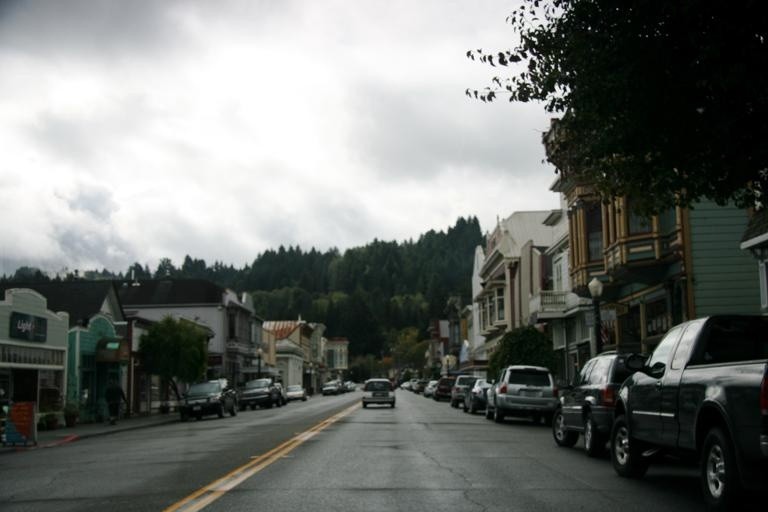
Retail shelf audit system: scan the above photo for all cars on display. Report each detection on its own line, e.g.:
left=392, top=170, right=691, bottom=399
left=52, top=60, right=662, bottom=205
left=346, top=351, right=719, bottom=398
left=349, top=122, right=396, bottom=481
left=361, top=378, right=395, bottom=408
left=399, top=364, right=557, bottom=427
left=175, top=378, right=356, bottom=405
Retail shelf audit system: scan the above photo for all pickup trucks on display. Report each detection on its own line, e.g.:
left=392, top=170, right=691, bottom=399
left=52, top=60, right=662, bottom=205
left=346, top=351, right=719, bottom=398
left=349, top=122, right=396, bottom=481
left=612, top=314, right=767, bottom=510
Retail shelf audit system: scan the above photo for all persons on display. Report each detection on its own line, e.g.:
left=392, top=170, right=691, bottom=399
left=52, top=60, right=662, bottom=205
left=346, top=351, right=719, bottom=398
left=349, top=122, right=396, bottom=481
left=103, top=378, right=129, bottom=424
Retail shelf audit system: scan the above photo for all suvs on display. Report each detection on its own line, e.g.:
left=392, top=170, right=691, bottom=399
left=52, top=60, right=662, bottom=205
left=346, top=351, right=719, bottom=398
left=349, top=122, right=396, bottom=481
left=546, top=350, right=650, bottom=456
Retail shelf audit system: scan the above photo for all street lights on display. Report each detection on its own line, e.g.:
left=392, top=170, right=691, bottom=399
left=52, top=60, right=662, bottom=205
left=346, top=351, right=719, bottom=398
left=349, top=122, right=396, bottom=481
left=308, top=362, right=312, bottom=394
left=256, top=347, right=262, bottom=377
left=586, top=275, right=605, bottom=353
left=445, top=354, right=450, bottom=377
